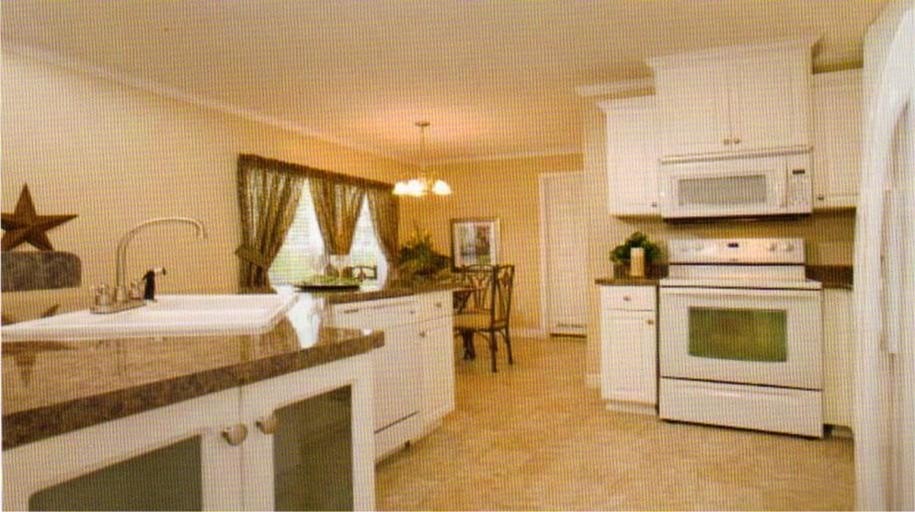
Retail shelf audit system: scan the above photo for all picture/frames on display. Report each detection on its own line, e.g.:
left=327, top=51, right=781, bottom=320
left=450, top=216, right=501, bottom=272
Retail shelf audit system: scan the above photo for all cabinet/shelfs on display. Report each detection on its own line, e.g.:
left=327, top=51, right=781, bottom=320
left=658, top=50, right=812, bottom=164
left=2, top=350, right=378, bottom=511
left=815, top=82, right=865, bottom=211
left=601, top=285, right=659, bottom=417
left=608, top=107, right=657, bottom=217
left=337, top=289, right=455, bottom=463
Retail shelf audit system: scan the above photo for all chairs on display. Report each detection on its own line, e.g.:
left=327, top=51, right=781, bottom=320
left=453, top=265, right=515, bottom=372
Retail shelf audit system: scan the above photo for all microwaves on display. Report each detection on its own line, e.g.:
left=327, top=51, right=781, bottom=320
left=661, top=152, right=814, bottom=220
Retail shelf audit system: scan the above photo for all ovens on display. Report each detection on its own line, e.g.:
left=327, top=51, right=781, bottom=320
left=660, top=281, right=826, bottom=441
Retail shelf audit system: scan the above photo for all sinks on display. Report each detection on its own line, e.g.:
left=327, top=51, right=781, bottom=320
left=2, top=292, right=300, bottom=336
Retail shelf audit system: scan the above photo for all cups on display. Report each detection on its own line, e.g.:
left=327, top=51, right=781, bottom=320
left=631, top=246, right=645, bottom=277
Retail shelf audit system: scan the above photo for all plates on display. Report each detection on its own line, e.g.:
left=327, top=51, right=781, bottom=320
left=293, top=283, right=360, bottom=291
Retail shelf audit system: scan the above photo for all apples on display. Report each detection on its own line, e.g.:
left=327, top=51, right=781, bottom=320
left=615, top=232, right=660, bottom=258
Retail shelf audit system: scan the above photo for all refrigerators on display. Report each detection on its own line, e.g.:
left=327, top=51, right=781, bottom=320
left=850, top=1, right=914, bottom=512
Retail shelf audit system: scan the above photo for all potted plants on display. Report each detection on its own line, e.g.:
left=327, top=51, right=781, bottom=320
left=608, top=232, right=661, bottom=277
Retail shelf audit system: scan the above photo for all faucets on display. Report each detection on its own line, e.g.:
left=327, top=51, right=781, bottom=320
left=114, top=215, right=209, bottom=303
